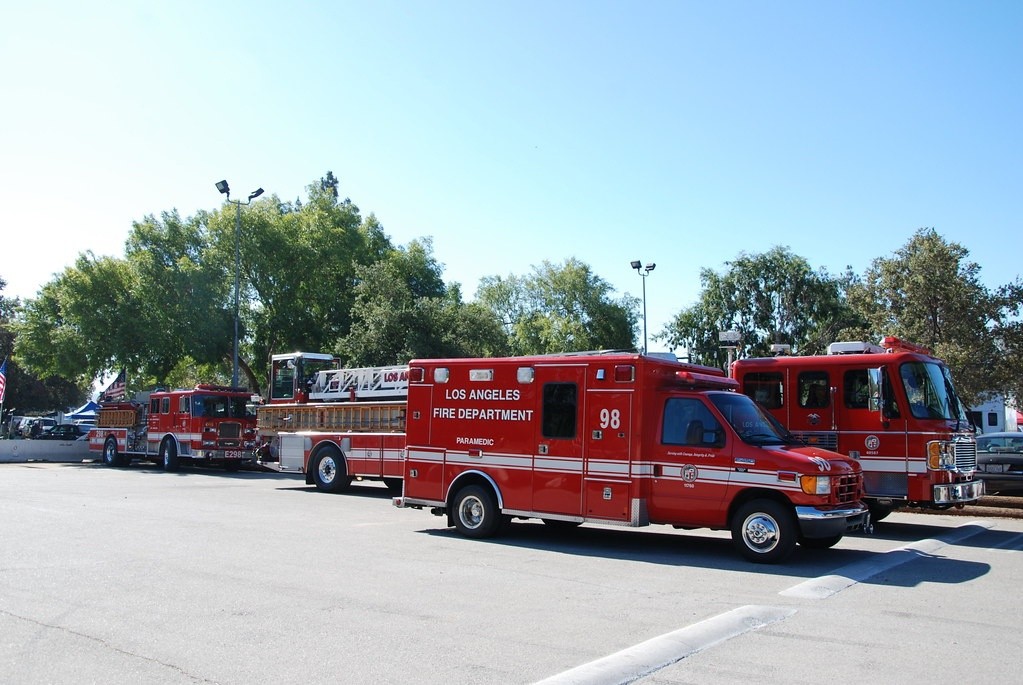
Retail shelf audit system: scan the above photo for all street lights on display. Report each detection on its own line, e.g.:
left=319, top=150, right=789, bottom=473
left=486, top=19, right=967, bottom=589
left=630, top=260, right=657, bottom=353
left=214, top=179, right=265, bottom=391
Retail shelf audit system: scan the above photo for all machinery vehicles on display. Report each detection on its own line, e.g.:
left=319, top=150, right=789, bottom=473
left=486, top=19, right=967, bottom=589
left=256, top=352, right=410, bottom=493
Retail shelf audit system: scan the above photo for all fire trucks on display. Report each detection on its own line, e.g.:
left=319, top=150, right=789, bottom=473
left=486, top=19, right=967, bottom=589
left=719, top=335, right=986, bottom=523
left=393, top=349, right=874, bottom=565
left=89, top=383, right=257, bottom=472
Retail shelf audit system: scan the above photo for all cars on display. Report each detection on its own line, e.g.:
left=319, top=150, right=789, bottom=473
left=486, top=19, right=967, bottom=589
left=18, top=418, right=96, bottom=440
left=975, top=432, right=1023, bottom=474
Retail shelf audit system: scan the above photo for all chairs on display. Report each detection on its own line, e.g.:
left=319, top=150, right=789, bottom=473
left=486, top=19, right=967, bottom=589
left=806, top=383, right=824, bottom=407
